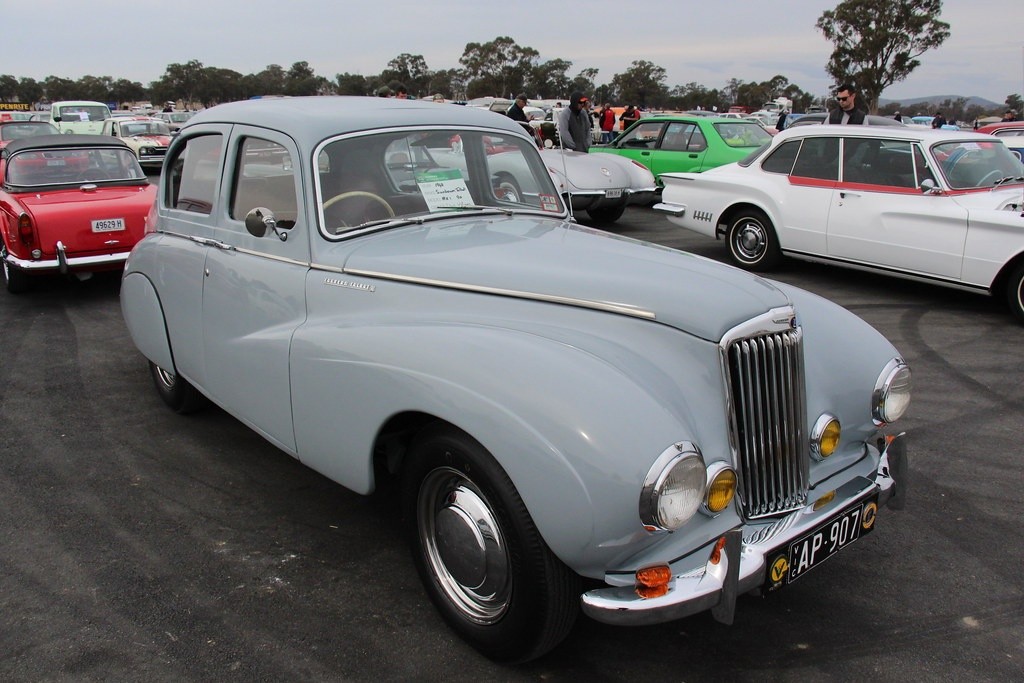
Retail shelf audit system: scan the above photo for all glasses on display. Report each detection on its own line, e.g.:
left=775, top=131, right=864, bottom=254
left=837, top=95, right=853, bottom=101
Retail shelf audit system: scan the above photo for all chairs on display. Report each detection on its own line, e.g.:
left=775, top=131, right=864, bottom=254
left=663, top=134, right=687, bottom=149
left=196, top=173, right=428, bottom=219
left=769, top=156, right=923, bottom=185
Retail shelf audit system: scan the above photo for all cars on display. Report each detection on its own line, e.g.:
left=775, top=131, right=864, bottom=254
left=0, top=100, right=293, bottom=179
left=116, top=93, right=915, bottom=670
left=586, top=113, right=774, bottom=193
left=650, top=123, right=1023, bottom=329
left=384, top=129, right=663, bottom=225
left=0, top=134, right=160, bottom=294
left=418, top=94, right=1024, bottom=171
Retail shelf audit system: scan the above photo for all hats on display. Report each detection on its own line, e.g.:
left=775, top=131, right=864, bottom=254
left=516, top=94, right=530, bottom=103
left=579, top=97, right=588, bottom=102
left=378, top=86, right=392, bottom=96
left=1011, top=110, right=1018, bottom=115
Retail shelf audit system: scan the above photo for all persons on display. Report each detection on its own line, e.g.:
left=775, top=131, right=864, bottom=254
left=619, top=104, right=641, bottom=139
left=395, top=86, right=407, bottom=99
left=163, top=103, right=173, bottom=112
left=932, top=112, right=946, bottom=129
left=974, top=117, right=980, bottom=129
left=506, top=93, right=539, bottom=148
left=599, top=103, right=615, bottom=144
left=379, top=86, right=391, bottom=98
left=894, top=111, right=901, bottom=122
left=823, top=84, right=868, bottom=124
left=1001, top=111, right=1017, bottom=122
left=557, top=92, right=596, bottom=154
left=776, top=110, right=788, bottom=131
